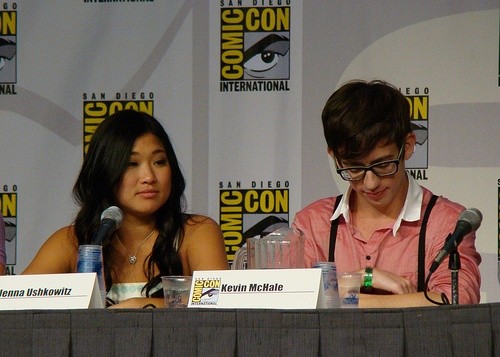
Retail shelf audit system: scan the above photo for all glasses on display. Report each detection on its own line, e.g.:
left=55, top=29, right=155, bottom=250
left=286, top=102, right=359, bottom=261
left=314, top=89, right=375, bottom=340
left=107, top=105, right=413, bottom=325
left=335, top=141, right=405, bottom=181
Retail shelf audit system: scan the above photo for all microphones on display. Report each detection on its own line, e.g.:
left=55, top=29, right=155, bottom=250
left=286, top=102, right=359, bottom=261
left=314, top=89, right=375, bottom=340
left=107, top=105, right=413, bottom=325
left=429, top=208, right=482, bottom=272
left=93, top=205, right=124, bottom=245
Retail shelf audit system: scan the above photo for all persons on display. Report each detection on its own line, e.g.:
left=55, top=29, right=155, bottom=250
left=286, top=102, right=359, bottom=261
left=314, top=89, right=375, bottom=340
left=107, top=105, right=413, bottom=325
left=291, top=80, right=482, bottom=308
left=19, top=110, right=230, bottom=309
left=0, top=213, right=7, bottom=275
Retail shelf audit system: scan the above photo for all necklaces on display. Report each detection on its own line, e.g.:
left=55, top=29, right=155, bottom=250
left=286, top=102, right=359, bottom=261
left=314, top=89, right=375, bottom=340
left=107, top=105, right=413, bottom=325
left=114, top=229, right=156, bottom=265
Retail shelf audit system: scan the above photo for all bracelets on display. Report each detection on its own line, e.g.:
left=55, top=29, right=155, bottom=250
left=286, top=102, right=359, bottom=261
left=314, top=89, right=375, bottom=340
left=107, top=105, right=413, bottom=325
left=364, top=267, right=373, bottom=287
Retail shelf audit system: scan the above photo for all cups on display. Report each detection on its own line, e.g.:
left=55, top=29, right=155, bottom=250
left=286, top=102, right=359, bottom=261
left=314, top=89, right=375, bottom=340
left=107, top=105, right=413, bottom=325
left=160, top=275, right=193, bottom=308
left=336, top=271, right=364, bottom=308
left=73, top=243, right=107, bottom=308
left=312, top=263, right=340, bottom=309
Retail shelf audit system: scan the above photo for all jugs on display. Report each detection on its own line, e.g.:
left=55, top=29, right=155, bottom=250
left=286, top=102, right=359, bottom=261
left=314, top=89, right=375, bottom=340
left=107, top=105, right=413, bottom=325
left=232, top=227, right=305, bottom=269
